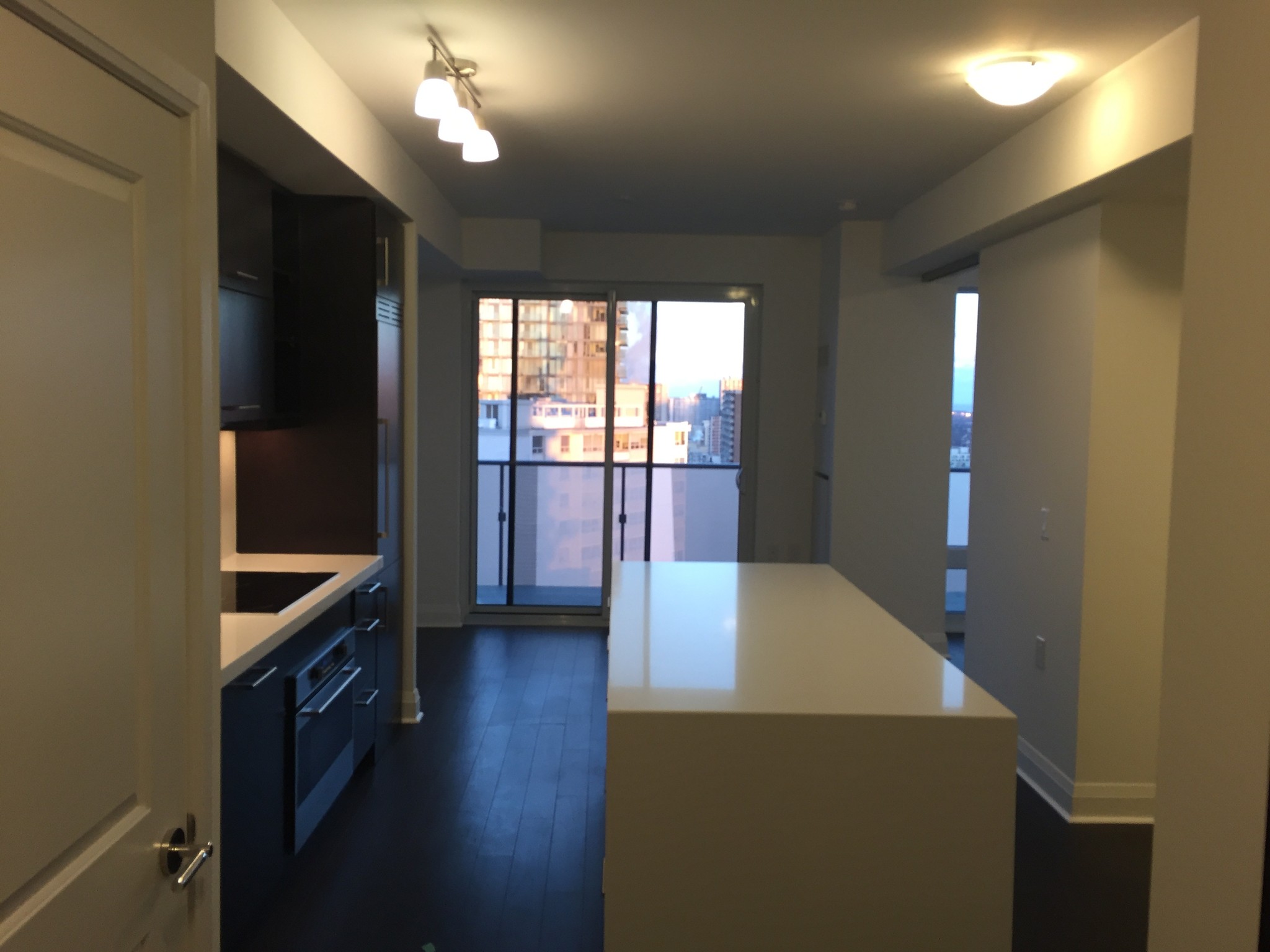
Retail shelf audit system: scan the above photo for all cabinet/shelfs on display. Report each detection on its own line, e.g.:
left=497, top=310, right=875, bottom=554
left=217, top=143, right=274, bottom=432
left=235, top=193, right=405, bottom=762
left=221, top=643, right=284, bottom=950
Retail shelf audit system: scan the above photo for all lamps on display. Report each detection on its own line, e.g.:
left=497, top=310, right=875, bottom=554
left=953, top=49, right=1080, bottom=106
left=414, top=36, right=500, bottom=162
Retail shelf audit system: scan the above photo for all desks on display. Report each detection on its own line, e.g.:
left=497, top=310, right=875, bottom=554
left=603, top=560, right=1015, bottom=952
